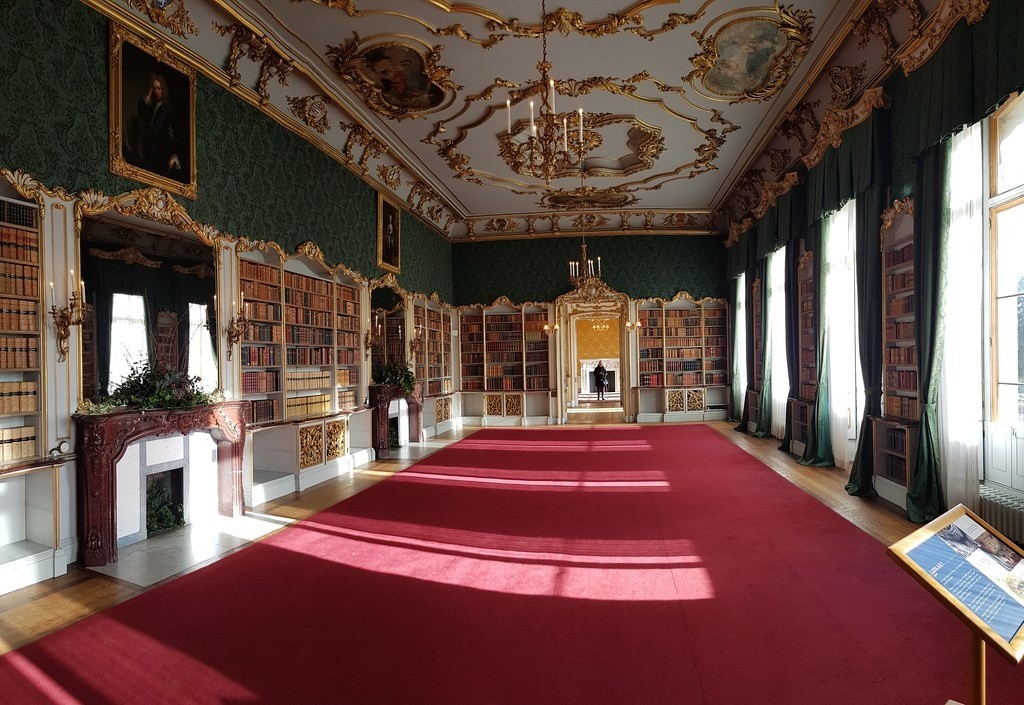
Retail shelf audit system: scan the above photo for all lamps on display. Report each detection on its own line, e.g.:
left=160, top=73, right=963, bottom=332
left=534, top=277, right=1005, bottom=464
left=567, top=170, right=604, bottom=295
left=364, top=315, right=383, bottom=362
left=48, top=268, right=96, bottom=363
left=497, top=0, right=592, bottom=188
left=625, top=320, right=641, bottom=334
left=588, top=318, right=610, bottom=332
left=543, top=323, right=560, bottom=337
left=397, top=325, right=405, bottom=354
left=203, top=294, right=217, bottom=338
left=409, top=324, right=423, bottom=362
left=227, top=291, right=248, bottom=362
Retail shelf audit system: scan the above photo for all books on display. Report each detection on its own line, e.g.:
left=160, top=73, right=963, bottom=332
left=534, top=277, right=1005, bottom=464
left=371, top=319, right=384, bottom=365
left=239, top=260, right=282, bottom=422
left=158, top=325, right=174, bottom=369
left=0, top=199, right=40, bottom=463
left=800, top=406, right=807, bottom=443
left=427, top=309, right=441, bottom=394
left=83, top=321, right=95, bottom=397
left=443, top=314, right=451, bottom=392
left=284, top=270, right=334, bottom=417
left=886, top=428, right=906, bottom=483
left=485, top=314, right=523, bottom=390
left=386, top=319, right=405, bottom=364
left=665, top=310, right=702, bottom=385
left=704, top=309, right=728, bottom=384
left=800, top=280, right=817, bottom=400
left=638, top=310, right=664, bottom=386
left=754, top=296, right=762, bottom=389
left=885, top=243, right=918, bottom=417
left=336, top=284, right=360, bottom=409
left=414, top=306, right=426, bottom=378
left=524, top=313, right=549, bottom=390
left=460, top=314, right=484, bottom=390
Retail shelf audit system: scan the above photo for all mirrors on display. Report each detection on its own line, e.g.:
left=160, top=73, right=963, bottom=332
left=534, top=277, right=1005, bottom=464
left=74, top=187, right=223, bottom=414
left=367, top=273, right=408, bottom=387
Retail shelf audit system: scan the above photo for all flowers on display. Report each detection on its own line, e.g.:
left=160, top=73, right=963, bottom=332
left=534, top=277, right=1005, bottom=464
left=78, top=303, right=229, bottom=415
left=372, top=356, right=418, bottom=399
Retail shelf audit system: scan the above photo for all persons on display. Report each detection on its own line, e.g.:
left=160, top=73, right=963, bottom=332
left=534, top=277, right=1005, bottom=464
left=593, top=360, right=607, bottom=401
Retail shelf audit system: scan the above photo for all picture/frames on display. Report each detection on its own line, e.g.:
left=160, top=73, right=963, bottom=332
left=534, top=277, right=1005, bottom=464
left=376, top=191, right=402, bottom=276
left=108, top=19, right=198, bottom=202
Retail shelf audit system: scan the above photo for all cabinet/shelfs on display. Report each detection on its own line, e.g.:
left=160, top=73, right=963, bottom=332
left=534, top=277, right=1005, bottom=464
left=702, top=297, right=731, bottom=421
left=484, top=295, right=523, bottom=427
left=871, top=196, right=920, bottom=513
left=282, top=241, right=352, bottom=493
left=387, top=301, right=405, bottom=363
left=636, top=297, right=664, bottom=422
left=746, top=277, right=762, bottom=434
left=80, top=303, right=99, bottom=405
left=441, top=301, right=454, bottom=433
left=157, top=310, right=179, bottom=373
left=407, top=292, right=436, bottom=439
left=237, top=235, right=296, bottom=509
left=334, top=261, right=372, bottom=471
left=426, top=291, right=443, bottom=436
left=0, top=195, right=55, bottom=599
left=664, top=291, right=704, bottom=422
left=790, top=251, right=815, bottom=458
left=523, top=301, right=552, bottom=425
left=372, top=307, right=387, bottom=364
left=457, top=303, right=485, bottom=426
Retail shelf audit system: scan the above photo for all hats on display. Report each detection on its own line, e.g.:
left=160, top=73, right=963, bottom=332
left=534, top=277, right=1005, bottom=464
left=599, top=360, right=603, bottom=365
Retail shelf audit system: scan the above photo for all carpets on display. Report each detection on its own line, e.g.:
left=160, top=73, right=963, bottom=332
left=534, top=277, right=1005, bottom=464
left=0, top=423, right=1024, bottom=705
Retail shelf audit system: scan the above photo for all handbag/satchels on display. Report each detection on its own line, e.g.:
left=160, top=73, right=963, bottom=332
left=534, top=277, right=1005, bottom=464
left=602, top=379, right=609, bottom=386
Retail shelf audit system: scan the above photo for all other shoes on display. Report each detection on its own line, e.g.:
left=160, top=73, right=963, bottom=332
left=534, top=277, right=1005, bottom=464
left=602, top=398, right=606, bottom=400
left=597, top=398, right=599, bottom=401
